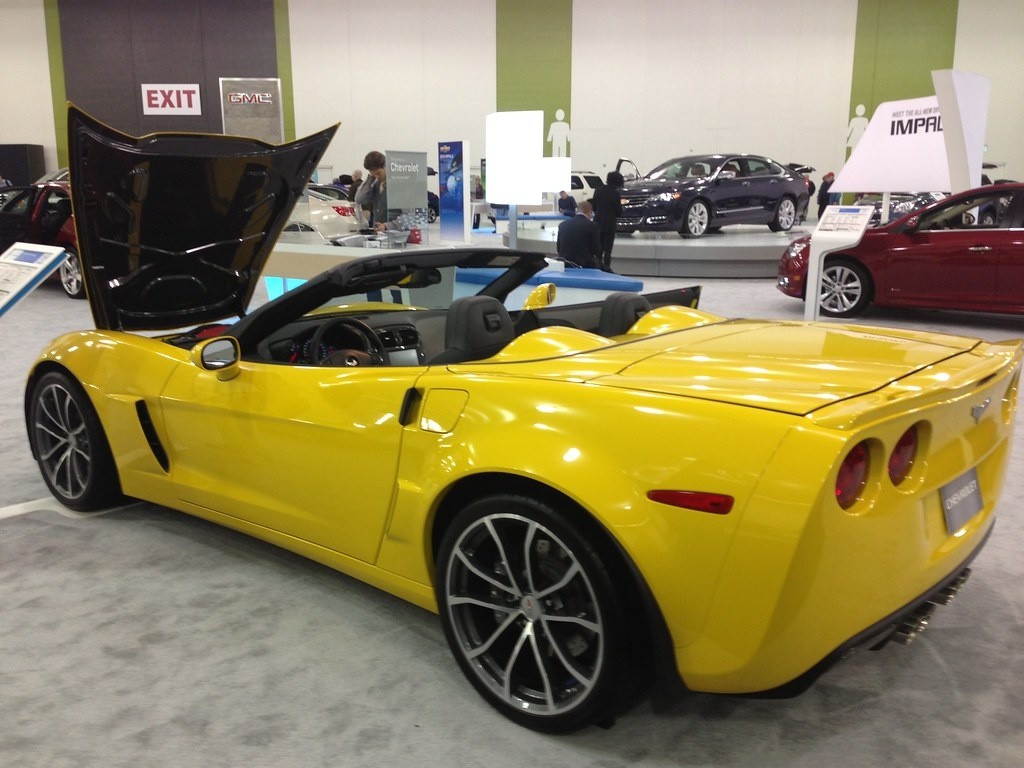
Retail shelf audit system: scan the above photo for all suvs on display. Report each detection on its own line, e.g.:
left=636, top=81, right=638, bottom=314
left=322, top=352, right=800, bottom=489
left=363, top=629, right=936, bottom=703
left=570, top=170, right=607, bottom=203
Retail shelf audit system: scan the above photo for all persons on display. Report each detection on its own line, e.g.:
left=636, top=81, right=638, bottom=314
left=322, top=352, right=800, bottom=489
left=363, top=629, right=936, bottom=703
left=557, top=171, right=624, bottom=274
left=803, top=172, right=841, bottom=222
left=348, top=151, right=416, bottom=234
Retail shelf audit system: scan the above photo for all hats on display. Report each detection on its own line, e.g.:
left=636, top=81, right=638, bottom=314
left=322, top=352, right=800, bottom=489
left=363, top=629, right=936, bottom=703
left=827, top=172, right=834, bottom=176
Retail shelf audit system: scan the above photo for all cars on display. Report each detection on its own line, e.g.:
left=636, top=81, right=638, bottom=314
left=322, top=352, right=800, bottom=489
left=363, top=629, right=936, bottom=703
left=776, top=165, right=1024, bottom=320
left=616, top=152, right=817, bottom=240
left=0, top=163, right=440, bottom=300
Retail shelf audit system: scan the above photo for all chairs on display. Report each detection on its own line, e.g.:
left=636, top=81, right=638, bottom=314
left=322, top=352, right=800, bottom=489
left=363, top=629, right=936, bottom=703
left=691, top=165, right=705, bottom=177
left=598, top=291, right=651, bottom=337
left=427, top=295, right=514, bottom=366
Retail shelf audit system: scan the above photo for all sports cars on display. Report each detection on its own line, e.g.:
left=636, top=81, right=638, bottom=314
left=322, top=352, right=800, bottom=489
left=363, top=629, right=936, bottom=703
left=24, top=100, right=996, bottom=735
